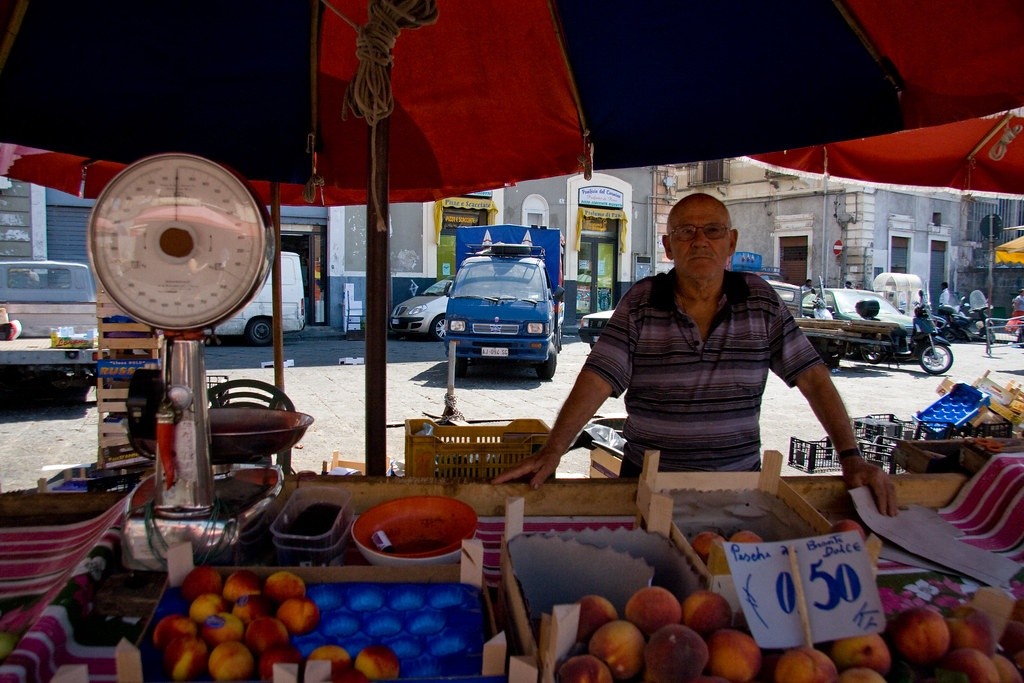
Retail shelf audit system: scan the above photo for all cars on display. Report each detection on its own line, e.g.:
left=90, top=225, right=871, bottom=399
left=577, top=309, right=615, bottom=349
left=390, top=275, right=456, bottom=340
left=816, top=287, right=914, bottom=364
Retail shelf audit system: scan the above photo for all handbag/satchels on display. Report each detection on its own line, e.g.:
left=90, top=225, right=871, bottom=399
left=1005, top=317, right=1023, bottom=333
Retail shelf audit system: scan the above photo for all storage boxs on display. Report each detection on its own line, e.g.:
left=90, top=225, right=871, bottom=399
left=39, top=289, right=1024, bottom=683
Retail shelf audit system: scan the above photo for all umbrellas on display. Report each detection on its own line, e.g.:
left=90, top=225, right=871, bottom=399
left=0, top=0, right=1024, bottom=481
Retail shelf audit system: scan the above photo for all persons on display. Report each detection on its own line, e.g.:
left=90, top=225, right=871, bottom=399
left=856, top=284, right=862, bottom=289
left=801, top=279, right=811, bottom=292
left=490, top=193, right=900, bottom=516
left=844, top=281, right=853, bottom=289
left=1014, top=289, right=1024, bottom=310
left=938, top=281, right=950, bottom=316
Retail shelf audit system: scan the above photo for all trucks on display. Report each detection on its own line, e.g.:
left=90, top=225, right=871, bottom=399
left=767, top=279, right=845, bottom=368
left=445, top=223, right=564, bottom=380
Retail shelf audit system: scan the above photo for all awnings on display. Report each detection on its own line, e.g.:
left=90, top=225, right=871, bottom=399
left=575, top=207, right=628, bottom=253
left=434, top=197, right=498, bottom=245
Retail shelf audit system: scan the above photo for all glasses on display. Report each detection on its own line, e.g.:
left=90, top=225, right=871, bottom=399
left=666, top=222, right=731, bottom=240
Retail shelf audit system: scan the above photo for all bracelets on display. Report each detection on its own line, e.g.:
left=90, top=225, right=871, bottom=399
left=836, top=446, right=862, bottom=464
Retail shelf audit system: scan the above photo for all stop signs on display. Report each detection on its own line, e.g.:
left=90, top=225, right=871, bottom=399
left=833, top=240, right=842, bottom=256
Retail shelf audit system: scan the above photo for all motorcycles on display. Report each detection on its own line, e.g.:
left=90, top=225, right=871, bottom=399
left=933, top=289, right=996, bottom=344
left=858, top=290, right=954, bottom=375
left=1014, top=313, right=1024, bottom=348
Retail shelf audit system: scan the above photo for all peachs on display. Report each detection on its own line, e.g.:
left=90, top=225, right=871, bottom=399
left=557, top=587, right=1024, bottom=683
left=151, top=566, right=400, bottom=683
left=831, top=519, right=865, bottom=540
left=692, top=530, right=763, bottom=563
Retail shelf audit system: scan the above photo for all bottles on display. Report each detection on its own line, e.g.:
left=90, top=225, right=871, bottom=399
left=51, top=328, right=73, bottom=348
left=88, top=329, right=98, bottom=348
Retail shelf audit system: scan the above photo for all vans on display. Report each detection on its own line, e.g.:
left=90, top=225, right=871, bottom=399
left=0, top=260, right=98, bottom=342
left=204, top=251, right=306, bottom=346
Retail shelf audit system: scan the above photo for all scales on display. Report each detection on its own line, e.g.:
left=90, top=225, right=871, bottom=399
left=86, top=151, right=314, bottom=566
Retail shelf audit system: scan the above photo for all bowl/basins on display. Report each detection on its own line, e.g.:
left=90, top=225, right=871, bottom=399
left=271, top=486, right=356, bottom=567
left=351, top=496, right=478, bottom=566
